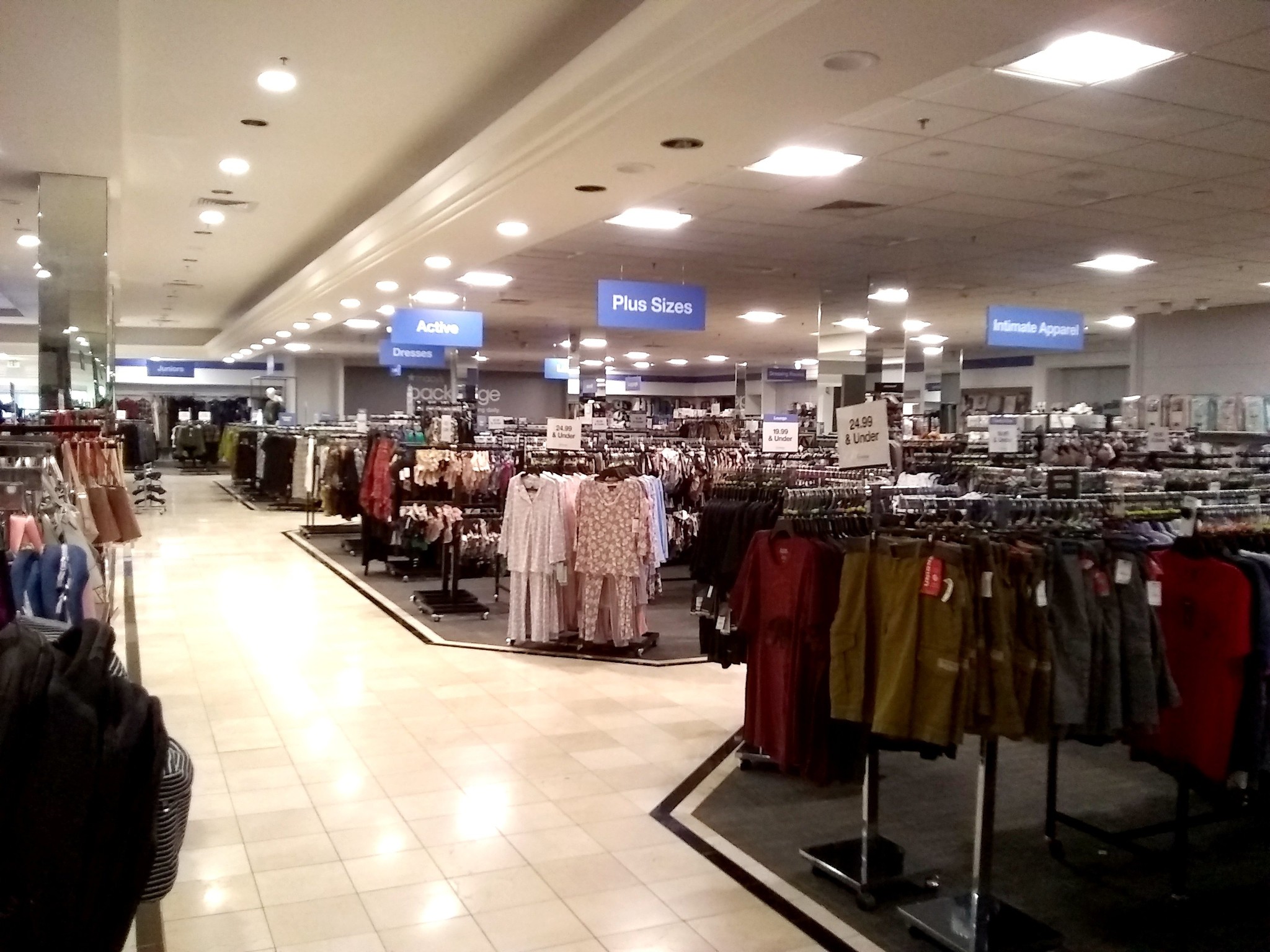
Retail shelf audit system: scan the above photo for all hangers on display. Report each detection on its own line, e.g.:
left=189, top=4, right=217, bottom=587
left=521, top=418, right=1270, bottom=552
left=124, top=393, right=130, bottom=402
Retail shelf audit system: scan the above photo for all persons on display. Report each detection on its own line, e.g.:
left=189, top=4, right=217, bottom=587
left=264, top=387, right=286, bottom=425
